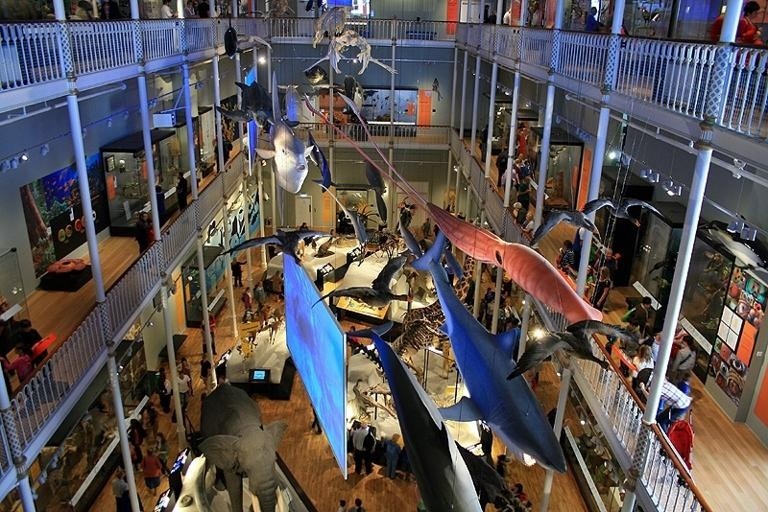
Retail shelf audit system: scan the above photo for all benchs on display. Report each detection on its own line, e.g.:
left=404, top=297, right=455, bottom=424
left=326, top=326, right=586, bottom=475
left=158, top=335, right=188, bottom=358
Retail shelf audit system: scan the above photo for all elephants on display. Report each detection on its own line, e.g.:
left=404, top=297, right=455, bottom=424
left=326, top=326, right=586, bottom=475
left=195, top=381, right=288, bottom=512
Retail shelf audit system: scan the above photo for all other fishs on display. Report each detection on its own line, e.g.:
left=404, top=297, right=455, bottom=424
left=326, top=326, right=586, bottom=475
left=397, top=214, right=422, bottom=260
left=212, top=64, right=364, bottom=194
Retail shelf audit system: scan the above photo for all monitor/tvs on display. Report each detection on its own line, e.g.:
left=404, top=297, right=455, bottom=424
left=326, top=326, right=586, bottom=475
left=248, top=367, right=271, bottom=383
left=170, top=447, right=190, bottom=475
left=152, top=488, right=172, bottom=512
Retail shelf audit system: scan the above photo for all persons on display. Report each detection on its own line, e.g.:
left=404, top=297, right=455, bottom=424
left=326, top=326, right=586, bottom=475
left=213, top=135, right=233, bottom=169
left=349, top=498, right=368, bottom=512
left=521, top=2, right=544, bottom=53
left=511, top=483, right=533, bottom=508
left=479, top=123, right=549, bottom=238
left=43, top=0, right=234, bottom=20
left=199, top=308, right=216, bottom=356
left=432, top=224, right=439, bottom=237
left=478, top=5, right=489, bottom=23
left=297, top=222, right=309, bottom=231
left=229, top=253, right=286, bottom=331
left=556, top=227, right=696, bottom=432
left=473, top=424, right=493, bottom=469
left=502, top=7, right=511, bottom=25
left=174, top=171, right=187, bottom=212
left=337, top=500, right=346, bottom=512
left=348, top=325, right=359, bottom=353
left=154, top=185, right=166, bottom=227
left=0, top=318, right=17, bottom=376
left=496, top=454, right=513, bottom=481
left=734, top=1, right=763, bottom=46
left=18, top=318, right=52, bottom=378
left=419, top=217, right=430, bottom=238
left=488, top=9, right=496, bottom=24
left=583, top=7, right=600, bottom=32
left=462, top=261, right=529, bottom=367
left=6, top=343, right=36, bottom=397
left=132, top=211, right=152, bottom=255
left=382, top=433, right=400, bottom=478
left=108, top=354, right=212, bottom=512
left=350, top=420, right=375, bottom=474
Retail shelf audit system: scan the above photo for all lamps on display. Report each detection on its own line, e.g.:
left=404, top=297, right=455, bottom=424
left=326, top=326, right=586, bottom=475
left=726, top=214, right=757, bottom=241
left=10, top=142, right=50, bottom=169
left=639, top=167, right=682, bottom=197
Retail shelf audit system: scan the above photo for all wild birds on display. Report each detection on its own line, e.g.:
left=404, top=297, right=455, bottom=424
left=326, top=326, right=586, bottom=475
left=505, top=319, right=639, bottom=382
left=216, top=225, right=330, bottom=266
left=529, top=197, right=616, bottom=247
left=580, top=190, right=677, bottom=230
left=308, top=255, right=413, bottom=308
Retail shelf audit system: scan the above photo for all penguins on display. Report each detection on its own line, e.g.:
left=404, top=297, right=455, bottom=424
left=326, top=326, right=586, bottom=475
left=698, top=214, right=768, bottom=271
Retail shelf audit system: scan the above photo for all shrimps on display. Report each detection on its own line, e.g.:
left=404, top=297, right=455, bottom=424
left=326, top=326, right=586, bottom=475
left=303, top=85, right=602, bottom=332
